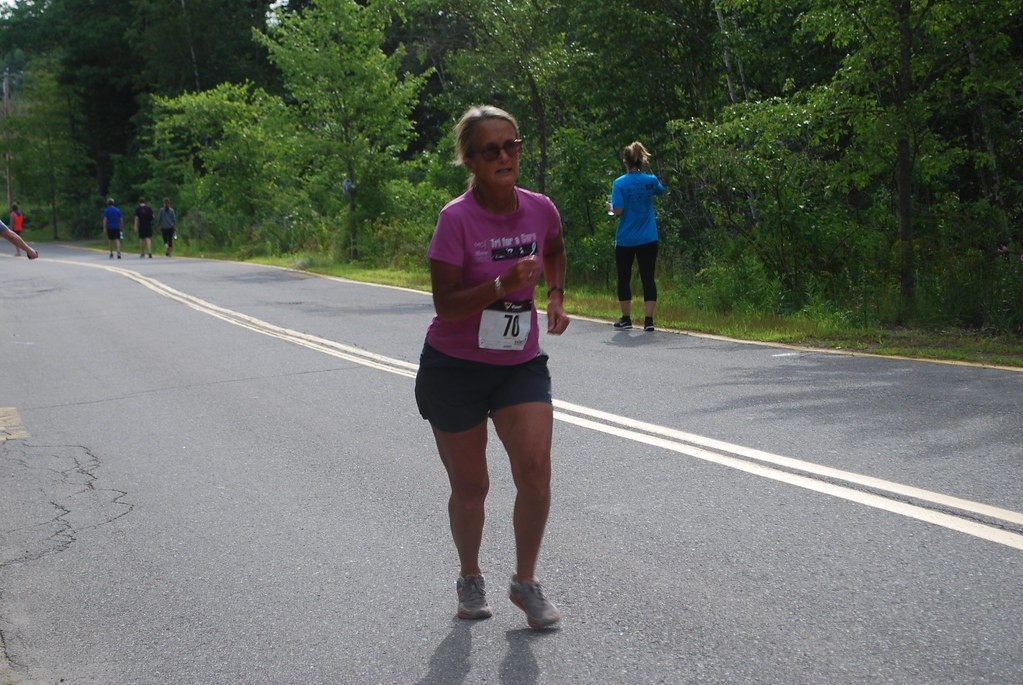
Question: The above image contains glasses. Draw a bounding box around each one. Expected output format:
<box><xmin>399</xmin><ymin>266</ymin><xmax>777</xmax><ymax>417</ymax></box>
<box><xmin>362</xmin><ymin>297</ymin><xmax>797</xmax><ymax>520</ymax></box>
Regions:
<box><xmin>471</xmin><ymin>139</ymin><xmax>523</xmax><ymax>161</ymax></box>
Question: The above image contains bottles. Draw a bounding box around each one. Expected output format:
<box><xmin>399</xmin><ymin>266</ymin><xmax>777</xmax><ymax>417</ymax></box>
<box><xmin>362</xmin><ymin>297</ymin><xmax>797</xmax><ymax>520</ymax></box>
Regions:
<box><xmin>120</xmin><ymin>231</ymin><xmax>123</xmax><ymax>239</ymax></box>
<box><xmin>606</xmin><ymin>195</ymin><xmax>614</xmax><ymax>215</ymax></box>
<box><xmin>174</xmin><ymin>231</ymin><xmax>177</xmax><ymax>239</ymax></box>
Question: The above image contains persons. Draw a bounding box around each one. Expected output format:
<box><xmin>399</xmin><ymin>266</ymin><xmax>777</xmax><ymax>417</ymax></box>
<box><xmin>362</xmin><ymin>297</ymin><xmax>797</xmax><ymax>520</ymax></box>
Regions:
<box><xmin>9</xmin><ymin>204</ymin><xmax>23</xmax><ymax>256</ymax></box>
<box><xmin>0</xmin><ymin>219</ymin><xmax>38</xmax><ymax>259</ymax></box>
<box><xmin>414</xmin><ymin>102</ymin><xmax>572</xmax><ymax>631</ymax></box>
<box><xmin>102</xmin><ymin>198</ymin><xmax>123</xmax><ymax>259</ymax></box>
<box><xmin>156</xmin><ymin>198</ymin><xmax>176</xmax><ymax>259</ymax></box>
<box><xmin>605</xmin><ymin>141</ymin><xmax>665</xmax><ymax>331</ymax></box>
<box><xmin>133</xmin><ymin>197</ymin><xmax>155</xmax><ymax>259</ymax></box>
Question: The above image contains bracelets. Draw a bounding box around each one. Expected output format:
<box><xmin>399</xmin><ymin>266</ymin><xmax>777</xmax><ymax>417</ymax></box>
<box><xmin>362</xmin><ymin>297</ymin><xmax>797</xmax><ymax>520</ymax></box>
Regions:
<box><xmin>547</xmin><ymin>286</ymin><xmax>564</xmax><ymax>298</ymax></box>
<box><xmin>495</xmin><ymin>275</ymin><xmax>506</xmax><ymax>298</ymax></box>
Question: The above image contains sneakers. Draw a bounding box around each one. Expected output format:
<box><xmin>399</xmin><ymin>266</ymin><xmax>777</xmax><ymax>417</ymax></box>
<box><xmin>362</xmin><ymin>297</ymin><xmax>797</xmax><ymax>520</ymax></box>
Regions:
<box><xmin>613</xmin><ymin>318</ymin><xmax>633</xmax><ymax>331</ymax></box>
<box><xmin>509</xmin><ymin>574</ymin><xmax>559</xmax><ymax>629</ymax></box>
<box><xmin>644</xmin><ymin>320</ymin><xmax>654</xmax><ymax>330</ymax></box>
<box><xmin>457</xmin><ymin>571</ymin><xmax>492</xmax><ymax>619</ymax></box>
